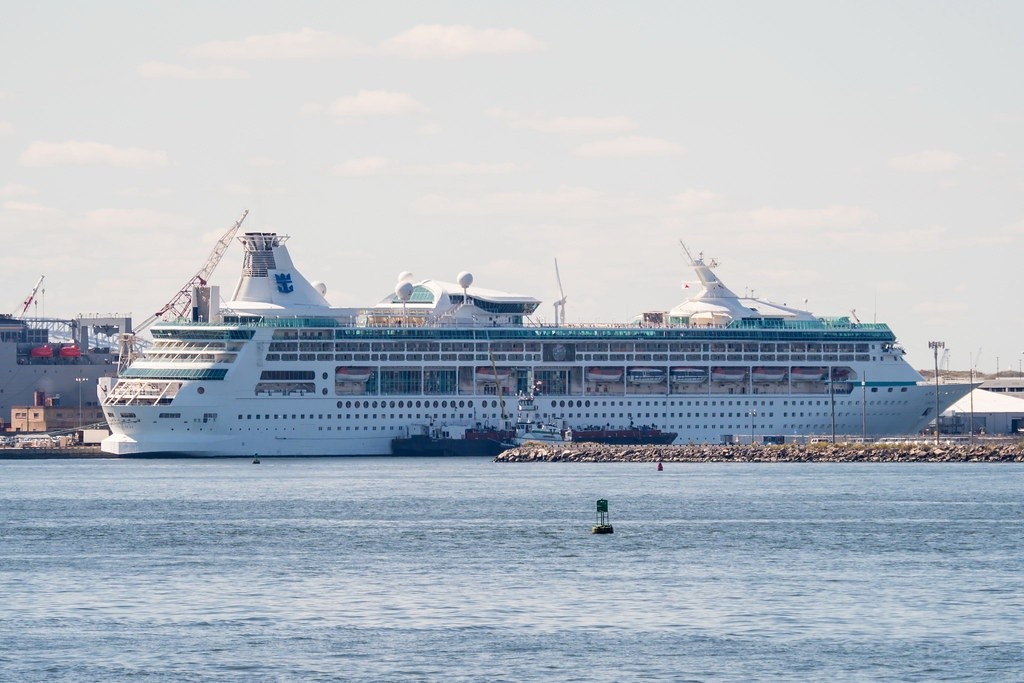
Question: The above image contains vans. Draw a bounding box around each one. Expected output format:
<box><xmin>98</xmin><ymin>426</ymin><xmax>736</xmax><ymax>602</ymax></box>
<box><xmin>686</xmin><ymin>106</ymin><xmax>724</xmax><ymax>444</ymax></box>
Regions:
<box><xmin>811</xmin><ymin>438</ymin><xmax>831</xmax><ymax>444</ymax></box>
<box><xmin>856</xmin><ymin>438</ymin><xmax>874</xmax><ymax>445</ymax></box>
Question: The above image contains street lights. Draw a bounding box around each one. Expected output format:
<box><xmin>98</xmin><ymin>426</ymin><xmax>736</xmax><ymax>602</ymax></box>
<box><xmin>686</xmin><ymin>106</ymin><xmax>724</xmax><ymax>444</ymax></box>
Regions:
<box><xmin>748</xmin><ymin>408</ymin><xmax>756</xmax><ymax>444</ymax></box>
<box><xmin>928</xmin><ymin>341</ymin><xmax>945</xmax><ymax>442</ymax></box>
<box><xmin>862</xmin><ymin>370</ymin><xmax>866</xmax><ymax>440</ymax></box>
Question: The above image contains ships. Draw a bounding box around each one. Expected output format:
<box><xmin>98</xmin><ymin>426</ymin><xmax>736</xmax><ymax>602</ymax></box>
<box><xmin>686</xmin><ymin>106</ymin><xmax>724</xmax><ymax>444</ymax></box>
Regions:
<box><xmin>0</xmin><ymin>274</ymin><xmax>131</xmax><ymax>427</ymax></box>
<box><xmin>95</xmin><ymin>209</ymin><xmax>987</xmax><ymax>458</ymax></box>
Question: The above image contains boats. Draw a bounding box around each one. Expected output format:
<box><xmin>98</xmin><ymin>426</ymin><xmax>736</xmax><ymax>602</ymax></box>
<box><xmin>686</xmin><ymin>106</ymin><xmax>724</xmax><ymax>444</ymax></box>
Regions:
<box><xmin>475</xmin><ymin>373</ymin><xmax>509</xmax><ymax>381</ymax></box>
<box><xmin>392</xmin><ymin>405</ymin><xmax>517</xmax><ymax>457</ymax></box>
<box><xmin>751</xmin><ymin>368</ymin><xmax>786</xmax><ymax>381</ymax></box>
<box><xmin>335</xmin><ymin>373</ymin><xmax>371</xmax><ymax>382</ymax></box>
<box><xmin>573</xmin><ymin>416</ymin><xmax>678</xmax><ymax>445</ymax></box>
<box><xmin>711</xmin><ymin>368</ymin><xmax>747</xmax><ymax>381</ymax></box>
<box><xmin>670</xmin><ymin>369</ymin><xmax>709</xmax><ymax>384</ymax></box>
<box><xmin>792</xmin><ymin>368</ymin><xmax>824</xmax><ymax>382</ymax></box>
<box><xmin>586</xmin><ymin>367</ymin><xmax>622</xmax><ymax>382</ymax></box>
<box><xmin>626</xmin><ymin>369</ymin><xmax>666</xmax><ymax>384</ymax></box>
<box><xmin>513</xmin><ymin>392</ymin><xmax>564</xmax><ymax>448</ymax></box>
